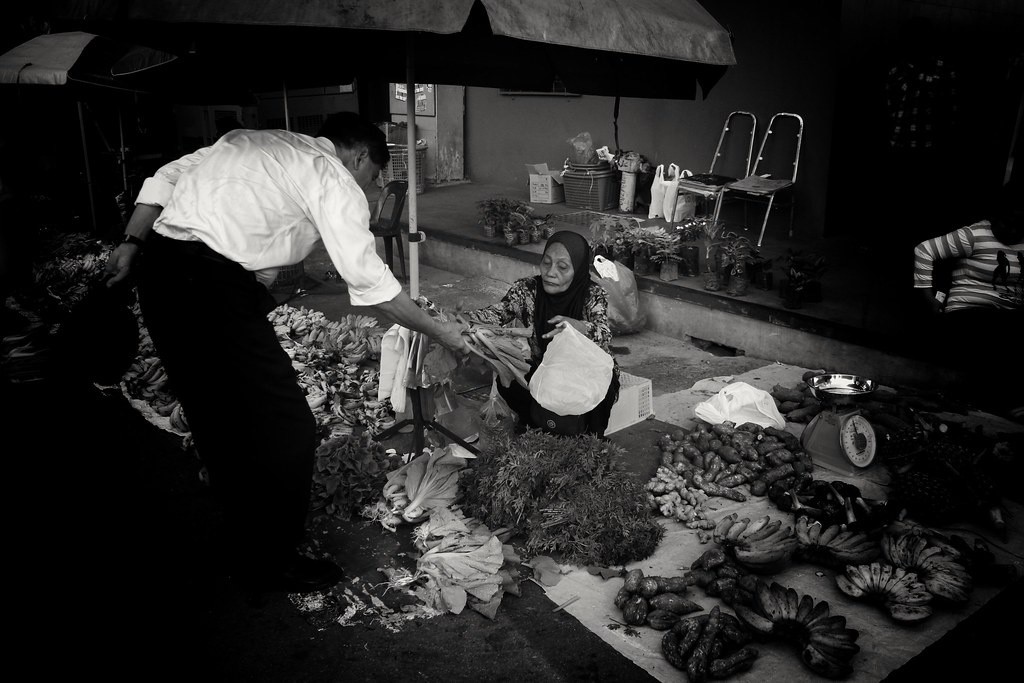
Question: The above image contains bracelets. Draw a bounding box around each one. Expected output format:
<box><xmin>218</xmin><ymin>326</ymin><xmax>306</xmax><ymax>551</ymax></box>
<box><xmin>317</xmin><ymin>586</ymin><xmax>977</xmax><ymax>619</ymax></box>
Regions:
<box><xmin>119</xmin><ymin>233</ymin><xmax>145</xmax><ymax>248</ymax></box>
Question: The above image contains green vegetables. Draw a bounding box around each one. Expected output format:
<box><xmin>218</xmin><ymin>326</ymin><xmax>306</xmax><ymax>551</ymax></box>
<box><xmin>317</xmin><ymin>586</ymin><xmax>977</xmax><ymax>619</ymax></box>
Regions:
<box><xmin>454</xmin><ymin>424</ymin><xmax>668</xmax><ymax>569</ymax></box>
<box><xmin>313</xmin><ymin>432</ymin><xmax>563</xmax><ymax>622</ymax></box>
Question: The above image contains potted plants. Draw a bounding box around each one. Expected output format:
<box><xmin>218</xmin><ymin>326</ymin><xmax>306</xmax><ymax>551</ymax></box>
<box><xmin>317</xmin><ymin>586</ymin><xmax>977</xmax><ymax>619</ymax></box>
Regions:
<box><xmin>475</xmin><ymin>199</ymin><xmax>556</xmax><ymax>247</ymax></box>
<box><xmin>588</xmin><ymin>215</ymin><xmax>827</xmax><ymax>310</ymax></box>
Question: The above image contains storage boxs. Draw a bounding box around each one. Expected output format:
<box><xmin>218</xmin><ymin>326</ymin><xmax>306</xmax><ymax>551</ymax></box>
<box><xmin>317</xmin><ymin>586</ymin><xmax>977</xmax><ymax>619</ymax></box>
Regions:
<box><xmin>604</xmin><ymin>370</ymin><xmax>653</xmax><ymax>436</ymax></box>
<box><xmin>560</xmin><ymin>159</ymin><xmax>620</xmax><ymax>211</ymax></box>
<box><xmin>524</xmin><ymin>163</ymin><xmax>565</xmax><ymax>204</ymax></box>
<box><xmin>377</xmin><ymin>143</ymin><xmax>428</xmax><ymax>197</ymax></box>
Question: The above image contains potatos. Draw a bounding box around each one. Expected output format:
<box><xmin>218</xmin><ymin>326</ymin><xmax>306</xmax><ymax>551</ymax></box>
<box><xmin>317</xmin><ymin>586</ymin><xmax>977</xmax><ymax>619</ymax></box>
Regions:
<box><xmin>658</xmin><ymin>420</ymin><xmax>814</xmax><ymax>502</ymax></box>
<box><xmin>662</xmin><ymin>604</ymin><xmax>759</xmax><ymax>682</ymax></box>
<box><xmin>614</xmin><ymin>568</ymin><xmax>704</xmax><ymax>631</ymax></box>
<box><xmin>770</xmin><ymin>371</ymin><xmax>824</xmax><ymax>423</ymax></box>
<box><xmin>683</xmin><ymin>549</ymin><xmax>761</xmax><ymax>595</ymax></box>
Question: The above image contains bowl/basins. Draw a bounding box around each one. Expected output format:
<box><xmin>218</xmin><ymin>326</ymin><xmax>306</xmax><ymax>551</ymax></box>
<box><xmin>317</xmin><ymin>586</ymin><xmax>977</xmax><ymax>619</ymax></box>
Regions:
<box><xmin>806</xmin><ymin>373</ymin><xmax>879</xmax><ymax>407</ymax></box>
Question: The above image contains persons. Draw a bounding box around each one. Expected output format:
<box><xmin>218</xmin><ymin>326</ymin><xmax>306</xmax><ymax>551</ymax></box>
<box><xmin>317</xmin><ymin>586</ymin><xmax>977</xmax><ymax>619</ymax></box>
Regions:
<box><xmin>100</xmin><ymin>117</ymin><xmax>472</xmax><ymax>610</ymax></box>
<box><xmin>412</xmin><ymin>231</ymin><xmax>621</xmax><ymax>442</ymax></box>
<box><xmin>913</xmin><ymin>180</ymin><xmax>1024</xmax><ymax>415</ymax></box>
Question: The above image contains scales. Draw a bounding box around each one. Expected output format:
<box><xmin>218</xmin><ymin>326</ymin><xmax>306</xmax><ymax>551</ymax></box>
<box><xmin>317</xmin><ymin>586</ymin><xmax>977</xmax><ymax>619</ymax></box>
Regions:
<box><xmin>798</xmin><ymin>371</ymin><xmax>889</xmax><ymax>477</ymax></box>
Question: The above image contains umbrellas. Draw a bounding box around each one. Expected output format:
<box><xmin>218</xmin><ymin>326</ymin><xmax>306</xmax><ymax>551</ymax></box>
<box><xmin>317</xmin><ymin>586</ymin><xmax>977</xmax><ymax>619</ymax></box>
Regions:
<box><xmin>0</xmin><ymin>30</ymin><xmax>219</xmax><ymax>232</ymax></box>
<box><xmin>82</xmin><ymin>0</ymin><xmax>738</xmax><ymax>456</ymax></box>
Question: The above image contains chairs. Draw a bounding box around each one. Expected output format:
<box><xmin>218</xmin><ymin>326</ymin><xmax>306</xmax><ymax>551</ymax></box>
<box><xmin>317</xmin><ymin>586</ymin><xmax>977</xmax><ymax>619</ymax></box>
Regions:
<box><xmin>336</xmin><ymin>180</ymin><xmax>409</xmax><ymax>284</ymax></box>
<box><xmin>669</xmin><ymin>111</ymin><xmax>756</xmax><ymax>234</ymax></box>
<box><xmin>714</xmin><ymin>113</ymin><xmax>803</xmax><ymax>246</ymax></box>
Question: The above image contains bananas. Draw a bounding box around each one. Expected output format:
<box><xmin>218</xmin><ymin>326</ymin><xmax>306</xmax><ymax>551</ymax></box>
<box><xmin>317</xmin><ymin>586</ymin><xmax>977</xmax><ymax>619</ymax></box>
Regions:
<box><xmin>264</xmin><ymin>303</ymin><xmax>397</xmax><ymax>438</ymax></box>
<box><xmin>713</xmin><ymin>512</ymin><xmax>972</xmax><ymax>676</ymax></box>
<box><xmin>124</xmin><ymin>355</ymin><xmax>190</xmax><ymax>432</ymax></box>
